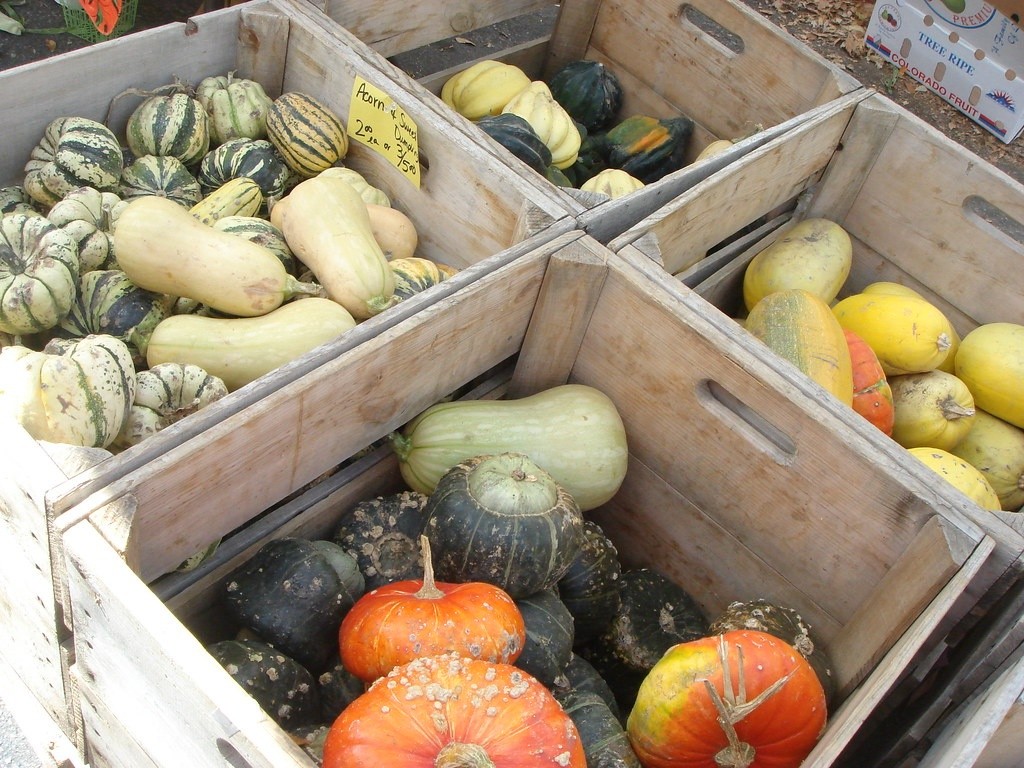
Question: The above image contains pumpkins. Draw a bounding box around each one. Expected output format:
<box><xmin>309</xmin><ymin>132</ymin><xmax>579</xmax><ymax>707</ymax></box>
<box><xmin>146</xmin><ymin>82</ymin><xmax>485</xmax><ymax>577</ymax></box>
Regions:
<box><xmin>0</xmin><ymin>57</ymin><xmax>1024</xmax><ymax>768</ymax></box>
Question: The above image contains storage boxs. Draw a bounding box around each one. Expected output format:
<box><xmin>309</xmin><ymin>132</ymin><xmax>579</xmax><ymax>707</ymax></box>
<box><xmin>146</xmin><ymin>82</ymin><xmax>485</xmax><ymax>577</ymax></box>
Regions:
<box><xmin>0</xmin><ymin>0</ymin><xmax>1024</xmax><ymax>768</ymax></box>
